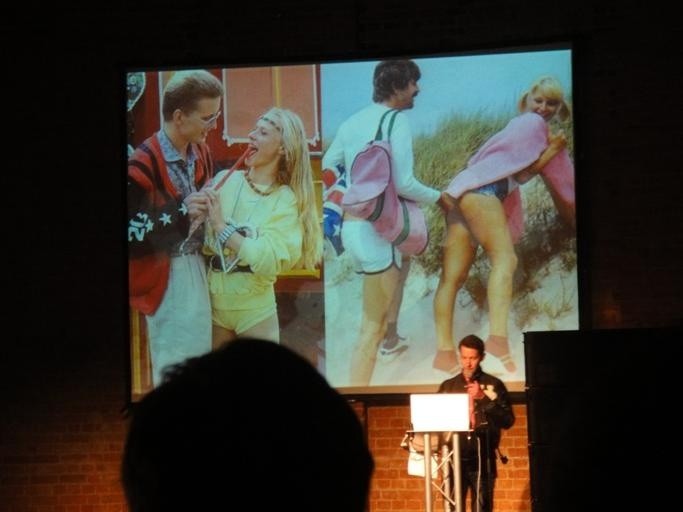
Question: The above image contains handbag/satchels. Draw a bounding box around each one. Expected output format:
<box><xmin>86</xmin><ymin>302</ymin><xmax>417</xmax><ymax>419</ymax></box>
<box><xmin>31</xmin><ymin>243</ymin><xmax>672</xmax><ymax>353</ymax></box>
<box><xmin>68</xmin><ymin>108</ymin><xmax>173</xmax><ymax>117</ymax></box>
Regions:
<box><xmin>338</xmin><ymin>108</ymin><xmax>433</xmax><ymax>257</ymax></box>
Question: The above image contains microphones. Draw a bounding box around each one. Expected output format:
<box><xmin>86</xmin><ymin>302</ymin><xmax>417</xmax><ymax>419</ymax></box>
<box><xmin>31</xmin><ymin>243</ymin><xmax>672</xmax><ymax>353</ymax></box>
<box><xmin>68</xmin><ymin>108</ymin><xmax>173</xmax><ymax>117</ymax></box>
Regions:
<box><xmin>462</xmin><ymin>367</ymin><xmax>474</xmax><ymax>383</ymax></box>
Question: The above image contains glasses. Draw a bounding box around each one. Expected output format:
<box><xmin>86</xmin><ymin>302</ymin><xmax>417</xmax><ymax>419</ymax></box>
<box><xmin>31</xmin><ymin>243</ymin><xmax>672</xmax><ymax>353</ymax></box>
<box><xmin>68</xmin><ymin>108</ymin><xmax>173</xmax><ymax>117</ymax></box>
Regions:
<box><xmin>194</xmin><ymin>111</ymin><xmax>223</xmax><ymax>128</ymax></box>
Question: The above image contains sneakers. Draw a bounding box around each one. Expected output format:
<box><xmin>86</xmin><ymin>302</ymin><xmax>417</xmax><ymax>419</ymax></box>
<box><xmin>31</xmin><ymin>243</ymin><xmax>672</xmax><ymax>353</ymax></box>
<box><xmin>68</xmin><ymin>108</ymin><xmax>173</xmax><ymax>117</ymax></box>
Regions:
<box><xmin>379</xmin><ymin>333</ymin><xmax>412</xmax><ymax>363</ymax></box>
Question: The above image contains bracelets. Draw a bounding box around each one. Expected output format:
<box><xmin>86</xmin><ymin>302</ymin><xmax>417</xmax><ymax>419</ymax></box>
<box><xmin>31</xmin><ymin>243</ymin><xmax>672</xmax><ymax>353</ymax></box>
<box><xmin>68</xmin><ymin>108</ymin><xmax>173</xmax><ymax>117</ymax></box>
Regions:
<box><xmin>218</xmin><ymin>224</ymin><xmax>237</xmax><ymax>245</ymax></box>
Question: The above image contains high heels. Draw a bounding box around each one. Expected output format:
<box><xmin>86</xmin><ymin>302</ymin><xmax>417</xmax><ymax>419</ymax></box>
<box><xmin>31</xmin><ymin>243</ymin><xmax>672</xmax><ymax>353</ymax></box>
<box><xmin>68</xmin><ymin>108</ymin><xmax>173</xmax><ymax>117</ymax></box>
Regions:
<box><xmin>478</xmin><ymin>346</ymin><xmax>525</xmax><ymax>380</ymax></box>
<box><xmin>431</xmin><ymin>363</ymin><xmax>465</xmax><ymax>384</ymax></box>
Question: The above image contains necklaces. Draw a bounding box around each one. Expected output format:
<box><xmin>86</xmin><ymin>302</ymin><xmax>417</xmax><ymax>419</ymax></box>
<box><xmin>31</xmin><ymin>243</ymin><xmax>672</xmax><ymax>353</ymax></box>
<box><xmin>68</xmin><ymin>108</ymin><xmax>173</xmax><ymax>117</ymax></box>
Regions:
<box><xmin>243</xmin><ymin>170</ymin><xmax>285</xmax><ymax>196</ymax></box>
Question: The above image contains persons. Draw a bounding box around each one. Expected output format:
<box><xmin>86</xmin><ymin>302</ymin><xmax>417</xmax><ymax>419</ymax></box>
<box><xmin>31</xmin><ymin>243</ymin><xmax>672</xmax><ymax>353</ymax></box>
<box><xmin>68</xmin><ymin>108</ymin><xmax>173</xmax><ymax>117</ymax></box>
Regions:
<box><xmin>436</xmin><ymin>335</ymin><xmax>514</xmax><ymax>512</ymax></box>
<box><xmin>128</xmin><ymin>69</ymin><xmax>224</xmax><ymax>388</ymax></box>
<box><xmin>202</xmin><ymin>109</ymin><xmax>323</xmax><ymax>351</ymax></box>
<box><xmin>120</xmin><ymin>338</ymin><xmax>373</xmax><ymax>512</ymax></box>
<box><xmin>322</xmin><ymin>59</ymin><xmax>457</xmax><ymax>387</ymax></box>
<box><xmin>433</xmin><ymin>78</ymin><xmax>574</xmax><ymax>374</ymax></box>
